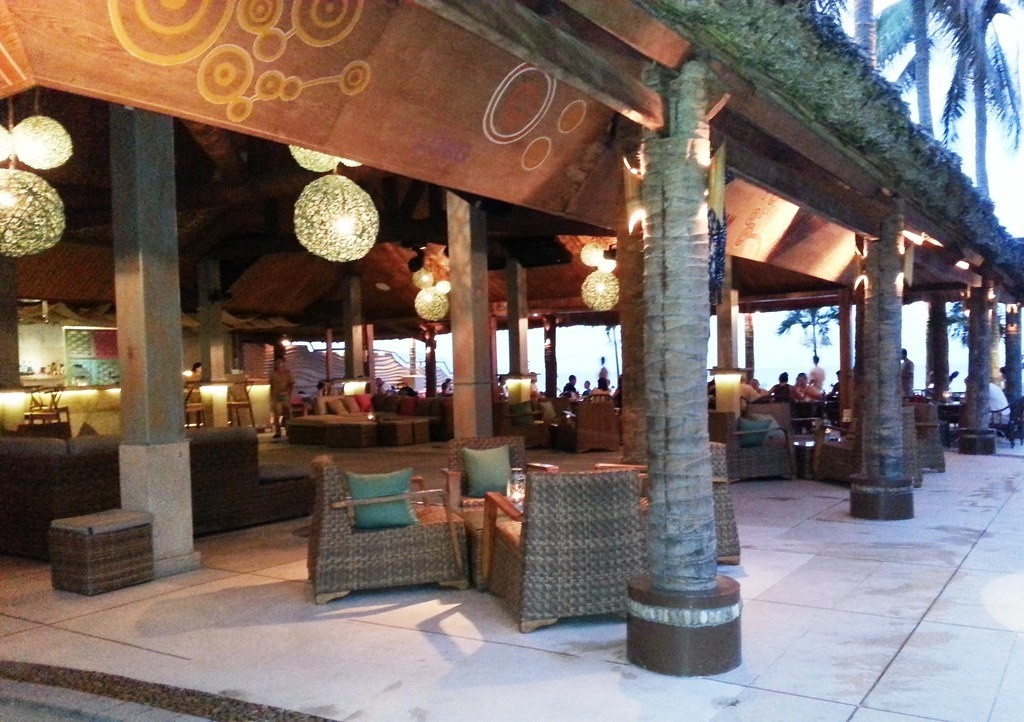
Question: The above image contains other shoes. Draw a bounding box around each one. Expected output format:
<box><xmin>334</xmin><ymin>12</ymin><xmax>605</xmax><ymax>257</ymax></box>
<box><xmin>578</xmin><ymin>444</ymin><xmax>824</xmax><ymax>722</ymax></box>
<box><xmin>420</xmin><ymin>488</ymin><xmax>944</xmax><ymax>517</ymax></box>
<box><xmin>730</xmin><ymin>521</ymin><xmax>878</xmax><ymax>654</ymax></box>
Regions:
<box><xmin>273</xmin><ymin>434</ymin><xmax>282</xmax><ymax>438</ymax></box>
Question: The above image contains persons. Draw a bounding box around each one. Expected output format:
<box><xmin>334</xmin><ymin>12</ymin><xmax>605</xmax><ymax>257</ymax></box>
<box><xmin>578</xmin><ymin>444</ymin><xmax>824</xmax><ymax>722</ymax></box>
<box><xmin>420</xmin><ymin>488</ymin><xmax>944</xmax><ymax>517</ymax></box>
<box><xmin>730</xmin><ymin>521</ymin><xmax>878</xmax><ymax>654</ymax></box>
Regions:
<box><xmin>563</xmin><ymin>373</ymin><xmax>577</xmax><ymax>393</ymax></box>
<box><xmin>596</xmin><ymin>367</ymin><xmax>622</xmax><ymax>408</ymax></box>
<box><xmin>963</xmin><ymin>375</ymin><xmax>1010</xmax><ymax>425</ymax></box>
<box><xmin>269</xmin><ymin>355</ymin><xmax>295</xmax><ymax>438</ymax></box>
<box><xmin>809</xmin><ymin>356</ymin><xmax>825</xmax><ymax>389</ymax></box>
<box><xmin>825</xmin><ymin>369</ymin><xmax>842</xmax><ymax>426</ymax></box>
<box><xmin>582</xmin><ymin>380</ymin><xmax>591</xmax><ymax>396</ymax></box>
<box><xmin>707</xmin><ymin>370</ymin><xmax>825</xmax><ymax>435</ymax></box>
<box><xmin>530</xmin><ymin>371</ymin><xmax>547</xmax><ymax>405</ymax></box>
<box><xmin>496</xmin><ymin>374</ymin><xmax>508</xmax><ymax>402</ymax></box>
<box><xmin>901</xmin><ymin>347</ymin><xmax>915</xmax><ymax>396</ymax></box>
<box><xmin>1000</xmin><ymin>366</ymin><xmax>1007</xmax><ymax>394</ymax></box>
<box><xmin>440</xmin><ymin>378</ymin><xmax>454</xmax><ymax>394</ymax></box>
<box><xmin>192</xmin><ymin>361</ymin><xmax>196</xmax><ymax>376</ymax></box>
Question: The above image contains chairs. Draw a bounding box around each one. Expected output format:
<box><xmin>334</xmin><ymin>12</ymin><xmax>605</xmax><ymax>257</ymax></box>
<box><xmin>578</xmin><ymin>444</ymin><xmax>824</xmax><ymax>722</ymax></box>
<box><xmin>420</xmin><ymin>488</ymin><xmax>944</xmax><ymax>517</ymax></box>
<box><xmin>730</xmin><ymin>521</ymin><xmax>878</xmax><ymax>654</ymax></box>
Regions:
<box><xmin>494</xmin><ymin>395</ymin><xmax>621</xmax><ymax>451</ymax></box>
<box><xmin>710</xmin><ymin>402</ymin><xmax>947</xmax><ymax>489</ymax></box>
<box><xmin>227</xmin><ymin>386</ymin><xmax>255</xmax><ymax>427</ymax></box>
<box><xmin>27</xmin><ymin>386</ymin><xmax>69</xmax><ymax>425</ymax></box>
<box><xmin>990</xmin><ymin>396</ymin><xmax>1024</xmax><ymax>448</ymax></box>
<box><xmin>185</xmin><ymin>386</ymin><xmax>206</xmax><ymax>427</ymax></box>
<box><xmin>310</xmin><ymin>439</ymin><xmax>743</xmax><ymax>631</ymax></box>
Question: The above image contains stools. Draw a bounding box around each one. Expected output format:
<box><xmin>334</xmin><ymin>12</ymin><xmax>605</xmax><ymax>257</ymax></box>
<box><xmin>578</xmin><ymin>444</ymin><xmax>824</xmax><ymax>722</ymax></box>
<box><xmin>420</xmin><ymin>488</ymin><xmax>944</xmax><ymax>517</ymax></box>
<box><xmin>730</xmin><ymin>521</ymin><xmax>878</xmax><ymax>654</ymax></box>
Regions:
<box><xmin>50</xmin><ymin>509</ymin><xmax>156</xmax><ymax>594</ymax></box>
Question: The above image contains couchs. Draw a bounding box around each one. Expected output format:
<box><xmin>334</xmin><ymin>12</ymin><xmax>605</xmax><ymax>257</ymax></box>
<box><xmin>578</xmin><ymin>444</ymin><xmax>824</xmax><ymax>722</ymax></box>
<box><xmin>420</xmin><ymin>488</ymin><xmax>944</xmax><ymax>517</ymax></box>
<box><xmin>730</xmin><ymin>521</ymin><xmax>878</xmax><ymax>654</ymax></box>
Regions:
<box><xmin>1</xmin><ymin>429</ymin><xmax>311</xmax><ymax>558</ymax></box>
<box><xmin>290</xmin><ymin>395</ymin><xmax>454</xmax><ymax>447</ymax></box>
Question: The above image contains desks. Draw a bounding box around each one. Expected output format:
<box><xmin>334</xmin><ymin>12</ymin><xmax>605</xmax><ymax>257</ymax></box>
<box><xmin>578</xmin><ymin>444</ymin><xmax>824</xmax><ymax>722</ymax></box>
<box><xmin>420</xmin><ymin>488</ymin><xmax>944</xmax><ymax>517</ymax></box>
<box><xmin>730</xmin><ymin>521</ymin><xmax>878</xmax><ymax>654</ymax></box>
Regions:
<box><xmin>941</xmin><ymin>401</ymin><xmax>966</xmax><ymax>443</ymax></box>
<box><xmin>459</xmin><ymin>510</ymin><xmax>489</xmax><ymax>590</ymax></box>
<box><xmin>790</xmin><ymin>439</ymin><xmax>815</xmax><ymax>478</ymax></box>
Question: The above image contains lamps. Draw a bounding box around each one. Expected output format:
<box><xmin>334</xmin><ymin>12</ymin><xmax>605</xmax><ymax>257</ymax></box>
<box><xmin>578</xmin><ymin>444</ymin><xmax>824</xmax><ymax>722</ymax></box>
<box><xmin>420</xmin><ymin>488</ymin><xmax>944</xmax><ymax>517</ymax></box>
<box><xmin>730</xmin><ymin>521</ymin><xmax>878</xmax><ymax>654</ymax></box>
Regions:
<box><xmin>410</xmin><ymin>270</ymin><xmax>451</xmax><ymax>320</ymax></box>
<box><xmin>285</xmin><ymin>140</ymin><xmax>380</xmax><ymax>262</ymax></box>
<box><xmin>508</xmin><ymin>475</ymin><xmax>526</xmax><ymax>507</ymax></box>
<box><xmin>581</xmin><ymin>243</ymin><xmax>620</xmax><ymax>309</ymax></box>
<box><xmin>0</xmin><ymin>115</ymin><xmax>73</xmax><ymax>257</ymax></box>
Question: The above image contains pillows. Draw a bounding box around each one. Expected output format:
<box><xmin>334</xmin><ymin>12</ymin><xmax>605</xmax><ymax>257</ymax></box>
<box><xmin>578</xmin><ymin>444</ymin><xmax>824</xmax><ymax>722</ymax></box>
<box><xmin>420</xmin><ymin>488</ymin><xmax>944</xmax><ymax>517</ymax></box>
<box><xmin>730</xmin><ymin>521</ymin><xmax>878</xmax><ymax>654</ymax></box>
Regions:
<box><xmin>459</xmin><ymin>446</ymin><xmax>510</xmax><ymax>496</ymax></box>
<box><xmin>510</xmin><ymin>400</ymin><xmax>533</xmax><ymax>426</ymax></box>
<box><xmin>329</xmin><ymin>396</ymin><xmax>441</xmax><ymax>415</ymax></box>
<box><xmin>343</xmin><ymin>469</ymin><xmax>419</xmax><ymax>529</ymax></box>
<box><xmin>553</xmin><ymin>429</ymin><xmax>577</xmax><ymax>450</ymax></box>
<box><xmin>740</xmin><ymin>416</ymin><xmax>770</xmax><ymax>448</ymax></box>
